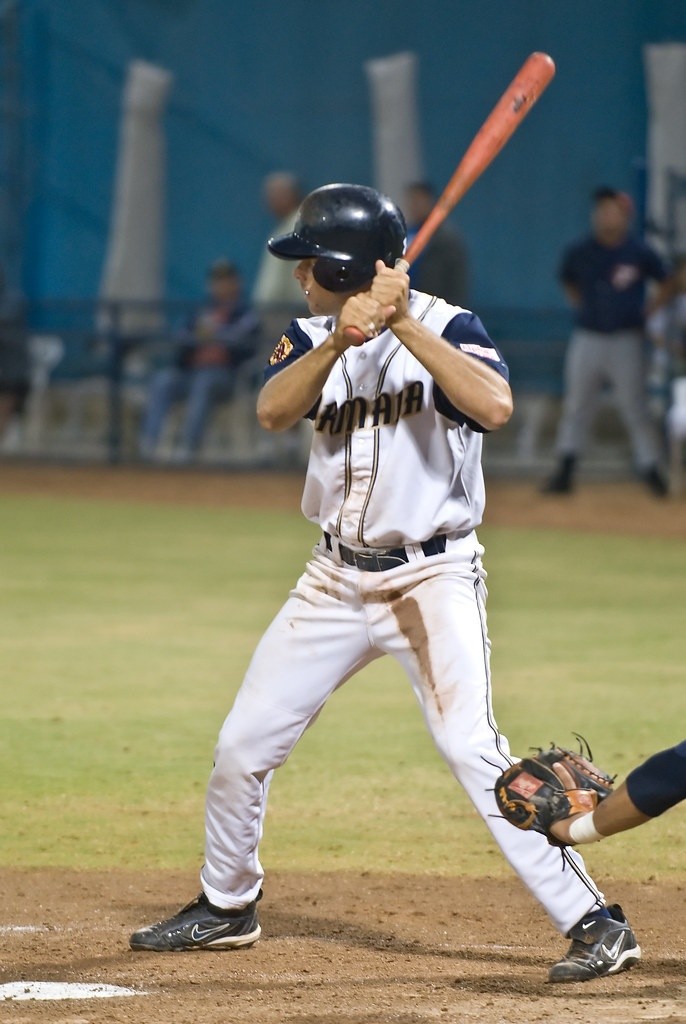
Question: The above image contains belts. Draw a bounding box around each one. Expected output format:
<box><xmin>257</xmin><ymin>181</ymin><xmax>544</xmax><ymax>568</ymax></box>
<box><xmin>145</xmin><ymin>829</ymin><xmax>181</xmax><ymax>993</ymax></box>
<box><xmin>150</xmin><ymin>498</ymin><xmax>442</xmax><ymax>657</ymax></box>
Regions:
<box><xmin>323</xmin><ymin>528</ymin><xmax>447</xmax><ymax>572</ymax></box>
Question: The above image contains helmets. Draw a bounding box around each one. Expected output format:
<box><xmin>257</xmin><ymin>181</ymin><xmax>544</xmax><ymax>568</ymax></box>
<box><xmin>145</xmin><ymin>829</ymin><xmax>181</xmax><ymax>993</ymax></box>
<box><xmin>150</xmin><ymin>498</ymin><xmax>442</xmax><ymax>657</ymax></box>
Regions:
<box><xmin>263</xmin><ymin>182</ymin><xmax>411</xmax><ymax>296</ymax></box>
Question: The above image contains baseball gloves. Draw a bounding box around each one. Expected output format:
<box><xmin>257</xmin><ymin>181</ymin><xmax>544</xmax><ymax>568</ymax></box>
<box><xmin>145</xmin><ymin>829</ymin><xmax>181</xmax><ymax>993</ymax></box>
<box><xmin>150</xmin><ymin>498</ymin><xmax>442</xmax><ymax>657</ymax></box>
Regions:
<box><xmin>492</xmin><ymin>748</ymin><xmax>616</xmax><ymax>847</ymax></box>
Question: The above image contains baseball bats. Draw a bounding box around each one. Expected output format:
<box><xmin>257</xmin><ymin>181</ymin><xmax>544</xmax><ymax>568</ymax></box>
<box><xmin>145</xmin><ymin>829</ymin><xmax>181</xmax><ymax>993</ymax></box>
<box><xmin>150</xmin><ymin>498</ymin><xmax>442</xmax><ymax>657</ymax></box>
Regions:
<box><xmin>342</xmin><ymin>47</ymin><xmax>554</xmax><ymax>347</ymax></box>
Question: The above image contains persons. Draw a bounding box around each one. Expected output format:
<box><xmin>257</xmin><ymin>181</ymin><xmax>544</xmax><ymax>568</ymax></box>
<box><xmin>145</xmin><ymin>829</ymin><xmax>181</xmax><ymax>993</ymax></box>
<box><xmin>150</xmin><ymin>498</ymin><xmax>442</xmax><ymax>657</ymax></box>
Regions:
<box><xmin>493</xmin><ymin>737</ymin><xmax>686</xmax><ymax>846</ymax></box>
<box><xmin>0</xmin><ymin>174</ymin><xmax>686</xmax><ymax>497</ymax></box>
<box><xmin>130</xmin><ymin>187</ymin><xmax>641</xmax><ymax>981</ymax></box>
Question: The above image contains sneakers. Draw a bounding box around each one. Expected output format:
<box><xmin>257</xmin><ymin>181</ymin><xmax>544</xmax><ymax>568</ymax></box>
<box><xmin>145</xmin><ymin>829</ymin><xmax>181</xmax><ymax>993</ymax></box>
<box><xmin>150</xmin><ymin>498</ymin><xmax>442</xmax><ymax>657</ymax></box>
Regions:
<box><xmin>546</xmin><ymin>904</ymin><xmax>642</xmax><ymax>985</ymax></box>
<box><xmin>129</xmin><ymin>888</ymin><xmax>265</xmax><ymax>953</ymax></box>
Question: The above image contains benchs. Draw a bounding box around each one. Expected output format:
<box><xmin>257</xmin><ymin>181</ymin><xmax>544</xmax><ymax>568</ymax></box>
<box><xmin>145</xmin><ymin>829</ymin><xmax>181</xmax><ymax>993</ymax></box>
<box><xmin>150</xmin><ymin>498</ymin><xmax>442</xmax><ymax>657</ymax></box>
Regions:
<box><xmin>28</xmin><ymin>334</ymin><xmax>312</xmax><ymax>471</ymax></box>
